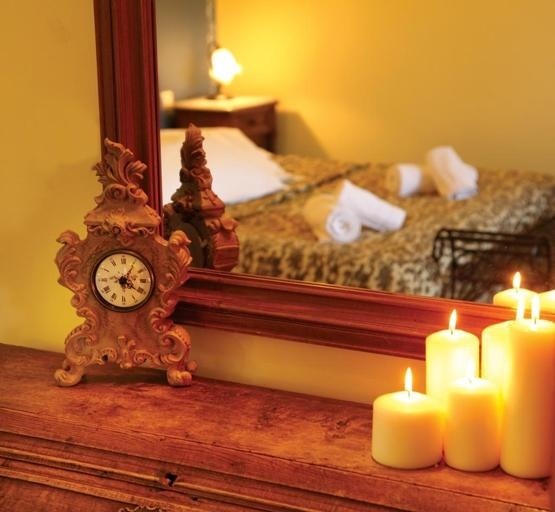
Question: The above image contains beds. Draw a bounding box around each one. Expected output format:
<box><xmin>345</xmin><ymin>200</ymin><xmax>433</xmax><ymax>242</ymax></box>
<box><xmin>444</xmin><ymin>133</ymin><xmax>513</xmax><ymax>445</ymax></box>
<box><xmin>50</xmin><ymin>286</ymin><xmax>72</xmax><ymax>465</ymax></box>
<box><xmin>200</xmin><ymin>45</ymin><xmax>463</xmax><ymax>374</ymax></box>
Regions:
<box><xmin>159</xmin><ymin>123</ymin><xmax>554</xmax><ymax>302</ymax></box>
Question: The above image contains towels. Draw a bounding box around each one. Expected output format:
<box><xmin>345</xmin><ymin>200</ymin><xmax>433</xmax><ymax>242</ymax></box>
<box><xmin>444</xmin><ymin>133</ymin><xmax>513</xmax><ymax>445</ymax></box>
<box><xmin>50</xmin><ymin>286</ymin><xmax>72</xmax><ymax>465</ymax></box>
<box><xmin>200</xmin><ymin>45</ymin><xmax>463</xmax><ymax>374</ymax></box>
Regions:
<box><xmin>304</xmin><ymin>195</ymin><xmax>362</xmax><ymax>244</ymax></box>
<box><xmin>429</xmin><ymin>145</ymin><xmax>478</xmax><ymax>201</ymax></box>
<box><xmin>384</xmin><ymin>163</ymin><xmax>477</xmax><ymax>197</ymax></box>
<box><xmin>332</xmin><ymin>178</ymin><xmax>406</xmax><ymax>232</ymax></box>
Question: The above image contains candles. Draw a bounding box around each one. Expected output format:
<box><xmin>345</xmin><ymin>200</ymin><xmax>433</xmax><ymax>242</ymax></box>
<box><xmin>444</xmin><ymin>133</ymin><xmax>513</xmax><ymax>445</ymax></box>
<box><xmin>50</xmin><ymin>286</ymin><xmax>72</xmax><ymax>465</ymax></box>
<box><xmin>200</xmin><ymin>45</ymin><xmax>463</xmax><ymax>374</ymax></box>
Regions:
<box><xmin>371</xmin><ymin>368</ymin><xmax>443</xmax><ymax>469</ymax></box>
<box><xmin>539</xmin><ymin>290</ymin><xmax>555</xmax><ymax>314</ymax></box>
<box><xmin>443</xmin><ymin>363</ymin><xmax>501</xmax><ymax>472</ymax></box>
<box><xmin>425</xmin><ymin>309</ymin><xmax>480</xmax><ymax>396</ymax></box>
<box><xmin>493</xmin><ymin>272</ymin><xmax>539</xmax><ymax>310</ymax></box>
<box><xmin>482</xmin><ymin>294</ymin><xmax>555</xmax><ymax>479</ymax></box>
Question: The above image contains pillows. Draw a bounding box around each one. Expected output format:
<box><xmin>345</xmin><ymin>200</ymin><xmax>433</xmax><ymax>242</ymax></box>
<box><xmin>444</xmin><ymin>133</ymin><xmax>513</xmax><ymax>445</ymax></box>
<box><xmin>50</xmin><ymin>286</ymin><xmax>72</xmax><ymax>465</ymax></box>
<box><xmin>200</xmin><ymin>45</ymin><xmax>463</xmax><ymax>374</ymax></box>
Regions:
<box><xmin>160</xmin><ymin>127</ymin><xmax>287</xmax><ymax>205</ymax></box>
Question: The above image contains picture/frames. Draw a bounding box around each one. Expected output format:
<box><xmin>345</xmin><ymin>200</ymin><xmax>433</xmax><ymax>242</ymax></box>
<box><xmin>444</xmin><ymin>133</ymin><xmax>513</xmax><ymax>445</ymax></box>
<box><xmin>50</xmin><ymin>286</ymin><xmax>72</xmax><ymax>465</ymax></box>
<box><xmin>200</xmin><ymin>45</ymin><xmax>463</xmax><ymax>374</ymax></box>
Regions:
<box><xmin>94</xmin><ymin>1</ymin><xmax>555</xmax><ymax>361</ymax></box>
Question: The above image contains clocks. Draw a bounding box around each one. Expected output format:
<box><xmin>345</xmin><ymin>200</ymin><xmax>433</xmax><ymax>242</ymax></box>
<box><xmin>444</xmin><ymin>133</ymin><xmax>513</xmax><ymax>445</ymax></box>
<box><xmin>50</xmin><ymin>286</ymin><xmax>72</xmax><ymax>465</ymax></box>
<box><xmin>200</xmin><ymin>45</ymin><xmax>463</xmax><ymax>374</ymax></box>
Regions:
<box><xmin>54</xmin><ymin>138</ymin><xmax>198</xmax><ymax>387</ymax></box>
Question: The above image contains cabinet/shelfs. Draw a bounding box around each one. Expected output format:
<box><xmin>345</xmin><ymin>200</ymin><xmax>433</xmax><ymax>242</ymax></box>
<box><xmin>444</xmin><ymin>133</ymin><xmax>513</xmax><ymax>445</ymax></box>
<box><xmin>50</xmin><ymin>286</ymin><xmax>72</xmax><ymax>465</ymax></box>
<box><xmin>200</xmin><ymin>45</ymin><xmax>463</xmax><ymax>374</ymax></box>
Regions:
<box><xmin>0</xmin><ymin>343</ymin><xmax>555</xmax><ymax>512</ymax></box>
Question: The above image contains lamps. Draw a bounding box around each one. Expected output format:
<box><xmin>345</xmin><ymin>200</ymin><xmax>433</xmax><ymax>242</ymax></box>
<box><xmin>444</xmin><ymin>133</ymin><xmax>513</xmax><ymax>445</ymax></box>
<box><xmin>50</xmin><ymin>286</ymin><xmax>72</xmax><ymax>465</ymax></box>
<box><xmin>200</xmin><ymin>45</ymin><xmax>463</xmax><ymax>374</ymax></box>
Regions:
<box><xmin>206</xmin><ymin>40</ymin><xmax>239</xmax><ymax>99</ymax></box>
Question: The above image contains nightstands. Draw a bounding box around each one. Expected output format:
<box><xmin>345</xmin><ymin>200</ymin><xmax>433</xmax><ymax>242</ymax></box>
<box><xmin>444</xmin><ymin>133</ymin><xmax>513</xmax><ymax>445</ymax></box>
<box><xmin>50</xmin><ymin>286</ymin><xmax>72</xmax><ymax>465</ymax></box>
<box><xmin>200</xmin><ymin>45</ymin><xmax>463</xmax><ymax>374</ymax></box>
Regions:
<box><xmin>173</xmin><ymin>97</ymin><xmax>279</xmax><ymax>154</ymax></box>
